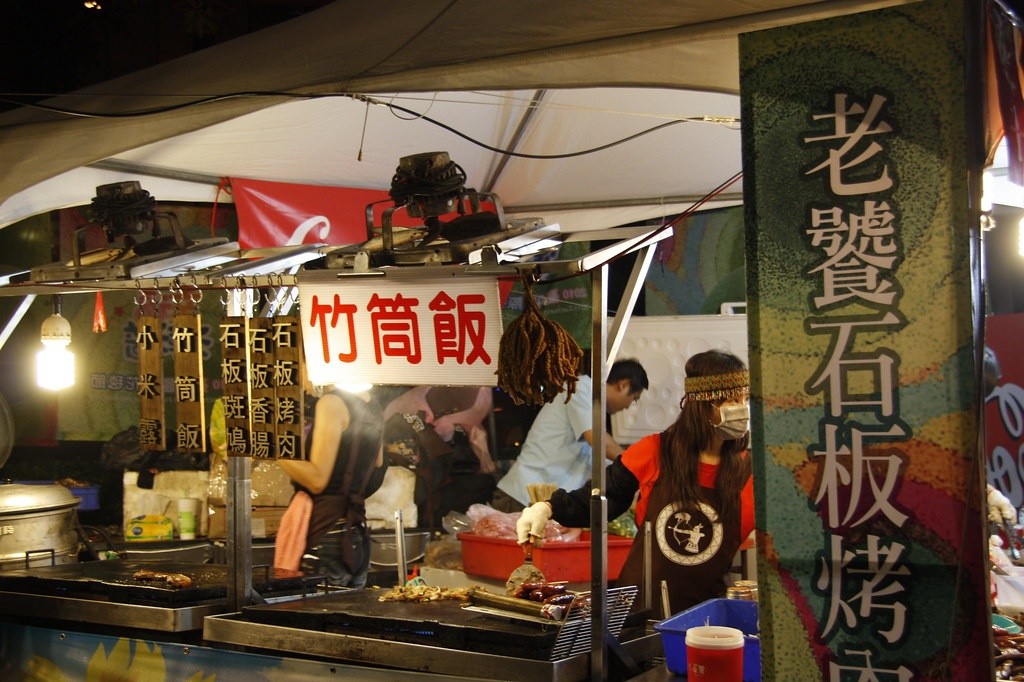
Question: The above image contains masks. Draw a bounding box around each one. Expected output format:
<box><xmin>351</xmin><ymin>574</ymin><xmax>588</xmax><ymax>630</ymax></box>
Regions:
<box><xmin>711</xmin><ymin>404</ymin><xmax>750</xmax><ymax>440</ymax></box>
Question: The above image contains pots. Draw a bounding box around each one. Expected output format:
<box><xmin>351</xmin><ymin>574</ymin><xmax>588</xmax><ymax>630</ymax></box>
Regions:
<box><xmin>0</xmin><ymin>479</ymin><xmax>85</xmax><ymax>571</ymax></box>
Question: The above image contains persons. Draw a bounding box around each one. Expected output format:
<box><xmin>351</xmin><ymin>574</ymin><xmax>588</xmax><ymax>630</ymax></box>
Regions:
<box><xmin>517</xmin><ymin>349</ymin><xmax>754</xmax><ymax>627</ymax></box>
<box><xmin>273</xmin><ymin>388</ymin><xmax>385</xmax><ymax>591</ymax></box>
<box><xmin>413</xmin><ymin>387</ymin><xmax>492</xmax><ymax>531</ymax></box>
<box><xmin>495</xmin><ymin>357</ymin><xmax>650</xmax><ymax>514</ymax></box>
<box><xmin>984</xmin><ymin>345</ymin><xmax>1016</xmax><ymax>529</ymax></box>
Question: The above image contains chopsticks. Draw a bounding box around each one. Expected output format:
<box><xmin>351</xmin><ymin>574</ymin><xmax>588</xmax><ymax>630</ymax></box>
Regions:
<box><xmin>528</xmin><ymin>484</ymin><xmax>557</xmax><ymax>502</ymax></box>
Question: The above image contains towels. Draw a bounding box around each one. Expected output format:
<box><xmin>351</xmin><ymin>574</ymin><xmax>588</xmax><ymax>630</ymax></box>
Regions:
<box><xmin>273</xmin><ymin>491</ymin><xmax>313</xmax><ymax>570</ymax></box>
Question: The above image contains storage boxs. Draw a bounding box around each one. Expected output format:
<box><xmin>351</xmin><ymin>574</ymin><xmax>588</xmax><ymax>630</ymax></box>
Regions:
<box><xmin>11</xmin><ymin>480</ymin><xmax>100</xmax><ymax>510</ymax></box>
<box><xmin>653</xmin><ymin>597</ymin><xmax>761</xmax><ymax>682</ymax></box>
<box><xmin>455</xmin><ymin>529</ymin><xmax>634</xmax><ymax>584</ymax></box>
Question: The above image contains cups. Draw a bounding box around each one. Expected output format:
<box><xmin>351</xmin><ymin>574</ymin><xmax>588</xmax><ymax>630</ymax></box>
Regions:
<box><xmin>177</xmin><ymin>498</ymin><xmax>197</xmax><ymax>541</ymax></box>
<box><xmin>686</xmin><ymin>626</ymin><xmax>745</xmax><ymax>682</ymax></box>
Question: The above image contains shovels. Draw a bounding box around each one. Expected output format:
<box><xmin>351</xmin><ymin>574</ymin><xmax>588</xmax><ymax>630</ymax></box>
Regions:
<box><xmin>506</xmin><ymin>537</ymin><xmax>548</xmax><ymax>595</ymax></box>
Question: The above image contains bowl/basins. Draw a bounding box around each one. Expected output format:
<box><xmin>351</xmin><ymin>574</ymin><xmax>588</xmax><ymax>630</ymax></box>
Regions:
<box><xmin>457</xmin><ymin>526</ymin><xmax>637</xmax><ymax>582</ymax></box>
<box><xmin>652</xmin><ymin>598</ymin><xmax>761</xmax><ymax>682</ymax></box>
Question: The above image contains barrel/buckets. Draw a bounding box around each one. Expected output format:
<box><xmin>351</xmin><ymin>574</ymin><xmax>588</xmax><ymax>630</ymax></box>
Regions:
<box><xmin>368</xmin><ymin>532</ymin><xmax>429</xmax><ymax>570</ymax></box>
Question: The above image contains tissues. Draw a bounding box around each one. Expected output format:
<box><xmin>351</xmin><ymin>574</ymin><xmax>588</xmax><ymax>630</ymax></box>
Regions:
<box><xmin>125</xmin><ymin>491</ymin><xmax>173</xmax><ymax>541</ymax></box>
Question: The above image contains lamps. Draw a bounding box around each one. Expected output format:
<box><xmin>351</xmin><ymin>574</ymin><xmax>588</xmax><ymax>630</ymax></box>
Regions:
<box><xmin>37</xmin><ymin>297</ymin><xmax>74</xmax><ymax>391</ymax></box>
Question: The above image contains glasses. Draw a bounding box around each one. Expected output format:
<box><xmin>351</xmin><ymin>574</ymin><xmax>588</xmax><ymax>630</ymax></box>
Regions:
<box><xmin>629</xmin><ymin>386</ymin><xmax>637</xmax><ymax>405</ymax></box>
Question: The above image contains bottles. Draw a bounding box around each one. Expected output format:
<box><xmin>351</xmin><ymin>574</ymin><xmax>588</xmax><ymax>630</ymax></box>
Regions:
<box><xmin>726</xmin><ymin>580</ymin><xmax>758</xmax><ymax>601</ymax></box>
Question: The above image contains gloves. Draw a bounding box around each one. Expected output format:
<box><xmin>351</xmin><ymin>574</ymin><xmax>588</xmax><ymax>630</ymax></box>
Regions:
<box><xmin>986</xmin><ymin>483</ymin><xmax>1017</xmax><ymax>531</ymax></box>
